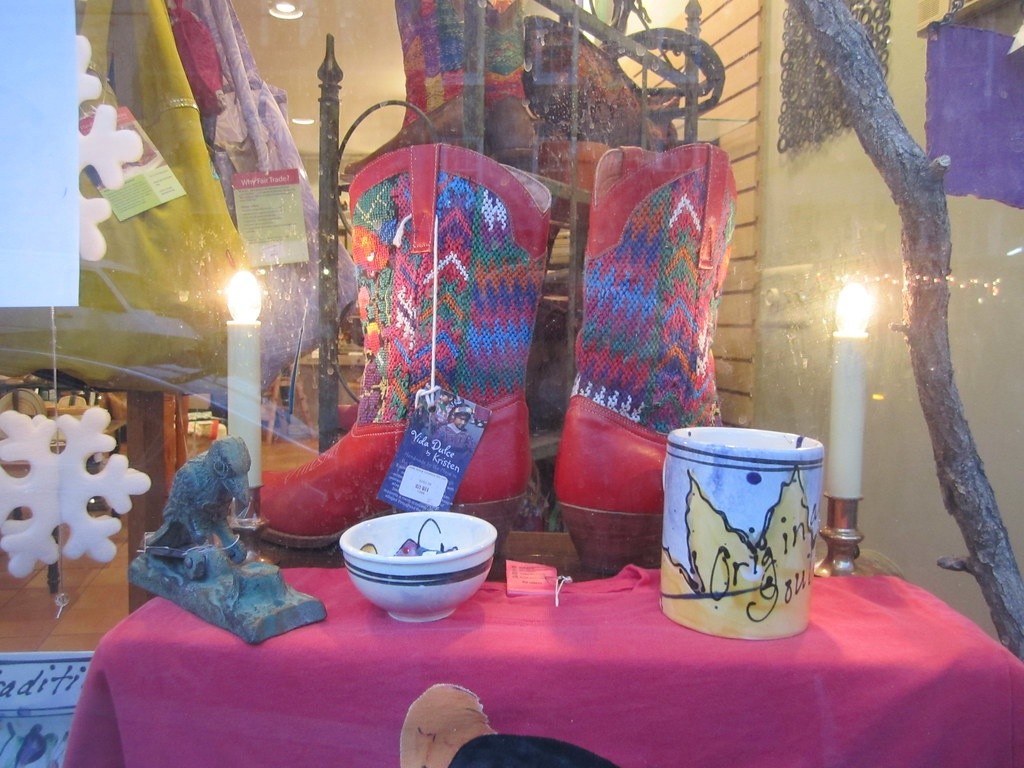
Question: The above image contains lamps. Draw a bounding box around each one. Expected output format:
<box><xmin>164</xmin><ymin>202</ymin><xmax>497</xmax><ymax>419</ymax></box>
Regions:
<box><xmin>825</xmin><ymin>281</ymin><xmax>875</xmax><ymax>497</ymax></box>
<box><xmin>225</xmin><ymin>269</ymin><xmax>262</xmax><ymax>486</ymax></box>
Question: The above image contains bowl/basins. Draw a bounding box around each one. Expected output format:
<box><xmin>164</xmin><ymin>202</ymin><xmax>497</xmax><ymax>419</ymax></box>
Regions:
<box><xmin>338</xmin><ymin>512</ymin><xmax>497</xmax><ymax>624</ymax></box>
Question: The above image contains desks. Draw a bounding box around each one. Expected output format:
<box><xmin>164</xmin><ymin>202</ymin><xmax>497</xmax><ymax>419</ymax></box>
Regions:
<box><xmin>61</xmin><ymin>533</ymin><xmax>1023</xmax><ymax>768</ymax></box>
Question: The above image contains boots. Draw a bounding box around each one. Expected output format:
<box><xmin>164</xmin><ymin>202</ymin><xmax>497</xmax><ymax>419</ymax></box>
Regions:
<box><xmin>555</xmin><ymin>143</ymin><xmax>736</xmax><ymax>574</ymax></box>
<box><xmin>258</xmin><ymin>143</ymin><xmax>550</xmax><ymax>548</ymax></box>
<box><xmin>340</xmin><ymin>0</ymin><xmax>539</xmax><ymax>182</ymax></box>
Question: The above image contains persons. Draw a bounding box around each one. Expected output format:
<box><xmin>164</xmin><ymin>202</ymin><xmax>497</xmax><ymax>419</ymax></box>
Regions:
<box><xmin>429</xmin><ymin>390</ymin><xmax>472</xmax><ymax>458</ymax></box>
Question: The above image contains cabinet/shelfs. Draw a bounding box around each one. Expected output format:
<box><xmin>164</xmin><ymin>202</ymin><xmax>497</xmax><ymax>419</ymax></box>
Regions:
<box><xmin>317</xmin><ymin>0</ymin><xmax>703</xmax><ymax>464</ymax></box>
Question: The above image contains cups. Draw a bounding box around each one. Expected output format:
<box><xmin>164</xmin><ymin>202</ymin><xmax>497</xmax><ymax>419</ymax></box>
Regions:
<box><xmin>657</xmin><ymin>426</ymin><xmax>824</xmax><ymax>640</ymax></box>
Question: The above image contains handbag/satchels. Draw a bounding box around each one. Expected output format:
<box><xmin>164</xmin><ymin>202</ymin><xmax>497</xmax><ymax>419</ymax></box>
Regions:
<box><xmin>1</xmin><ymin>0</ymin><xmax>360</xmax><ymax>392</ymax></box>
